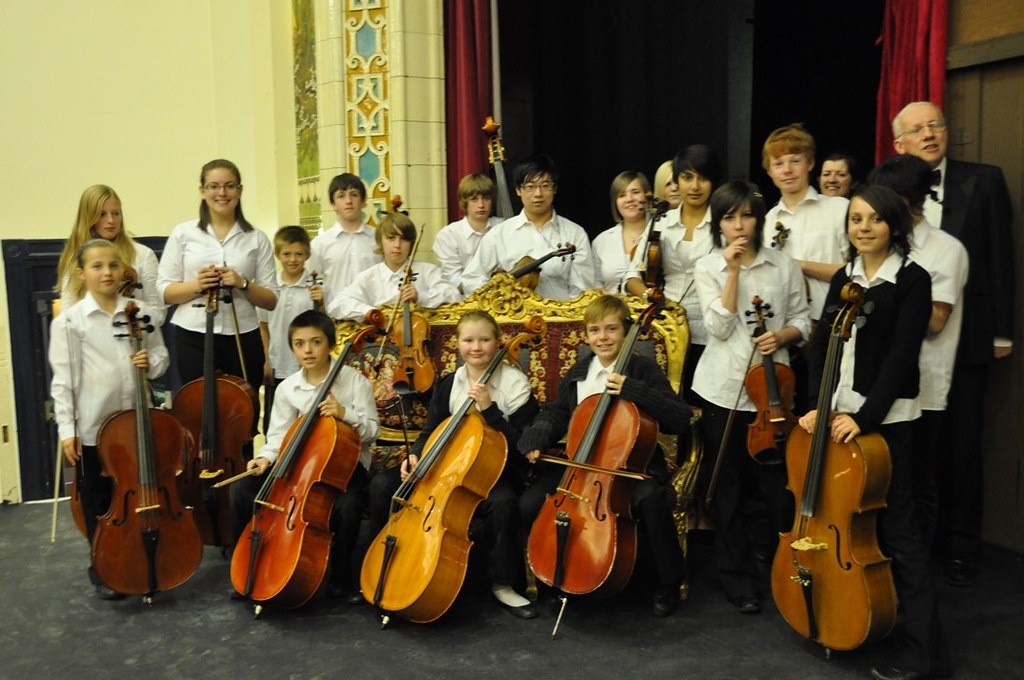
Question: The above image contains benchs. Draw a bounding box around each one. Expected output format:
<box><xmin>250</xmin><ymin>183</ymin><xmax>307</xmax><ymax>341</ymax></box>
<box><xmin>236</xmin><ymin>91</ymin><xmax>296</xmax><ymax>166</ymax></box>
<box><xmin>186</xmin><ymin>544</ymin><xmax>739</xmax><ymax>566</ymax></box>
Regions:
<box><xmin>329</xmin><ymin>268</ymin><xmax>702</xmax><ymax>601</ymax></box>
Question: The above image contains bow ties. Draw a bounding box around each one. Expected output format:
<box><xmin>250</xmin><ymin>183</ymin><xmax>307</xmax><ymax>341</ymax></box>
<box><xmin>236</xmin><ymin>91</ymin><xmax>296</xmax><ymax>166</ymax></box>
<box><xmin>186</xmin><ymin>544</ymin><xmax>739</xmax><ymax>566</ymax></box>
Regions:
<box><xmin>932</xmin><ymin>169</ymin><xmax>941</xmax><ymax>186</ymax></box>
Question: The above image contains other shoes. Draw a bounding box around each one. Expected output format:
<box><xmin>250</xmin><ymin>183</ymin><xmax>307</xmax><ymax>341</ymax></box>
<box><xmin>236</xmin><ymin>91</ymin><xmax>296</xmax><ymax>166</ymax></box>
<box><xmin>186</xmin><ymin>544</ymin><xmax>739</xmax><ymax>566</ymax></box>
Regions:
<box><xmin>870</xmin><ymin>662</ymin><xmax>932</xmax><ymax>680</ymax></box>
<box><xmin>736</xmin><ymin>592</ymin><xmax>761</xmax><ymax>612</ymax></box>
<box><xmin>489</xmin><ymin>587</ymin><xmax>538</xmax><ymax>618</ymax></box>
<box><xmin>654</xmin><ymin>589</ymin><xmax>675</xmax><ymax>617</ymax></box>
<box><xmin>753</xmin><ymin>546</ymin><xmax>769</xmax><ymax>562</ymax></box>
<box><xmin>346</xmin><ymin>590</ymin><xmax>363</xmax><ymax>603</ymax></box>
<box><xmin>96</xmin><ymin>582</ymin><xmax>126</xmax><ymax>600</ymax></box>
<box><xmin>946</xmin><ymin>551</ymin><xmax>978</xmax><ymax>587</ymax></box>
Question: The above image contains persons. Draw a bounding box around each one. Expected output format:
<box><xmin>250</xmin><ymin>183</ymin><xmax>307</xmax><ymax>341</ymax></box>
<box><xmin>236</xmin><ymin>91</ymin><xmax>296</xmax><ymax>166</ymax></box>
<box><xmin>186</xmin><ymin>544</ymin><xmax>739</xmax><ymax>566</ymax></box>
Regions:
<box><xmin>48</xmin><ymin>238</ymin><xmax>170</xmax><ymax>601</ymax></box>
<box><xmin>515</xmin><ymin>295</ymin><xmax>692</xmax><ymax>616</ymax></box>
<box><xmin>155</xmin><ymin>158</ymin><xmax>280</xmax><ymax>402</ymax></box>
<box><xmin>51</xmin><ymin>183</ymin><xmax>168</xmax><ymax>329</ymax></box>
<box><xmin>691</xmin><ymin>178</ymin><xmax>813</xmax><ymax>612</ymax></box>
<box><xmin>245</xmin><ymin>101</ymin><xmax>1017</xmax><ymax>680</ymax></box>
<box><xmin>431</xmin><ymin>172</ymin><xmax>507</xmax><ymax>295</ymax></box>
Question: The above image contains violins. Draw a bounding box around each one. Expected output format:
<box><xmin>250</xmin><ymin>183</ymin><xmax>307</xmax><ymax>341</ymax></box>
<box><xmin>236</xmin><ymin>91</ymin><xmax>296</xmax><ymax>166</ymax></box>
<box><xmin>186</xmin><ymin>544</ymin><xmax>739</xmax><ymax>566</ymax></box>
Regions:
<box><xmin>386</xmin><ymin>265</ymin><xmax>436</xmax><ymax>394</ymax></box>
<box><xmin>744</xmin><ymin>296</ymin><xmax>807</xmax><ymax>468</ymax></box>
<box><xmin>376</xmin><ymin>195</ymin><xmax>410</xmax><ymax>216</ymax></box>
<box><xmin>307</xmin><ymin>271</ymin><xmax>325</xmax><ymax>311</ymax></box>
<box><xmin>644</xmin><ymin>200</ymin><xmax>670</xmax><ymax>287</ymax></box>
<box><xmin>772</xmin><ymin>220</ymin><xmax>811</xmax><ymax>304</ymax></box>
<box><xmin>490</xmin><ymin>242</ymin><xmax>576</xmax><ymax>288</ymax></box>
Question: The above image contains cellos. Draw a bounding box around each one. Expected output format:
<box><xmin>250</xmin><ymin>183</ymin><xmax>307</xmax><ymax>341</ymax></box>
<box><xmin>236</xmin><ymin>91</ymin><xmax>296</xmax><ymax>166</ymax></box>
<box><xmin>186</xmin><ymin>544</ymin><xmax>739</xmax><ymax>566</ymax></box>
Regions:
<box><xmin>529</xmin><ymin>284</ymin><xmax>666</xmax><ymax>640</ymax></box>
<box><xmin>230</xmin><ymin>308</ymin><xmax>387</xmax><ymax>619</ymax></box>
<box><xmin>773</xmin><ymin>283</ymin><xmax>893</xmax><ymax>662</ymax></box>
<box><xmin>89</xmin><ymin>301</ymin><xmax>204</xmax><ymax>609</ymax></box>
<box><xmin>70</xmin><ymin>265</ymin><xmax>144</xmax><ymax>542</ymax></box>
<box><xmin>173</xmin><ymin>265</ymin><xmax>260</xmax><ymax>546</ymax></box>
<box><xmin>360</xmin><ymin>315</ymin><xmax>548</xmax><ymax>630</ymax></box>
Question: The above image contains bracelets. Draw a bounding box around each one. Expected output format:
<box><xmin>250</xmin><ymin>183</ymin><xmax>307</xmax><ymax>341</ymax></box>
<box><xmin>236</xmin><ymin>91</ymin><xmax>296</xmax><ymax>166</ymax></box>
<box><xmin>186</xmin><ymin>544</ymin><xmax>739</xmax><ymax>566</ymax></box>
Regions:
<box><xmin>239</xmin><ymin>276</ymin><xmax>249</xmax><ymax>292</ymax></box>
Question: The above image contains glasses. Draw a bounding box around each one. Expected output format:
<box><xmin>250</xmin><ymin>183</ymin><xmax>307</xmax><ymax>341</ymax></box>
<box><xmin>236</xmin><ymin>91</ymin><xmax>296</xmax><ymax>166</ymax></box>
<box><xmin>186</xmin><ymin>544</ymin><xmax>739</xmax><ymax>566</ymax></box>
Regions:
<box><xmin>897</xmin><ymin>121</ymin><xmax>947</xmax><ymax>137</ymax></box>
<box><xmin>202</xmin><ymin>181</ymin><xmax>241</xmax><ymax>193</ymax></box>
<box><xmin>521</xmin><ymin>182</ymin><xmax>554</xmax><ymax>192</ymax></box>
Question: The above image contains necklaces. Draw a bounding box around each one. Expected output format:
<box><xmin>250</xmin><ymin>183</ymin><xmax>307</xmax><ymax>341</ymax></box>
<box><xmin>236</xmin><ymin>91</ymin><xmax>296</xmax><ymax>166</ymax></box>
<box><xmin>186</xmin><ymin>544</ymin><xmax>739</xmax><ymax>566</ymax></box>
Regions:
<box><xmin>219</xmin><ymin>239</ymin><xmax>224</xmax><ymax>243</ymax></box>
